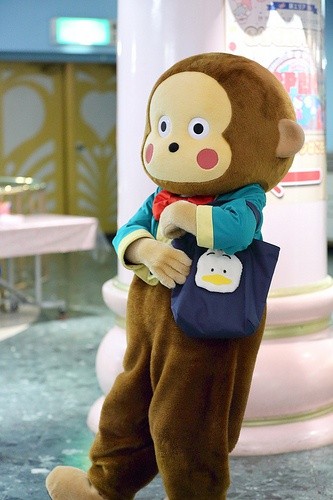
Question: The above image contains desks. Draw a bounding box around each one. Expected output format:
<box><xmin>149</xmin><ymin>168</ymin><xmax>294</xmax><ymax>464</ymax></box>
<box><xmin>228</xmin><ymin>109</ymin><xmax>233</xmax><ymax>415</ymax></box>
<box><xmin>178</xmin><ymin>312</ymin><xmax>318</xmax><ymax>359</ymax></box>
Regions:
<box><xmin>0</xmin><ymin>215</ymin><xmax>107</xmax><ymax>319</ymax></box>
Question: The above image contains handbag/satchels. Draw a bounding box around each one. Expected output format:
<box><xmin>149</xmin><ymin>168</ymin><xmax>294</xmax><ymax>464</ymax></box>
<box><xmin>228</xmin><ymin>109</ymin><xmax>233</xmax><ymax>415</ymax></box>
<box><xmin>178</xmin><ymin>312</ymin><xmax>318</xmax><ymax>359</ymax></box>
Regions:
<box><xmin>168</xmin><ymin>199</ymin><xmax>280</xmax><ymax>340</ymax></box>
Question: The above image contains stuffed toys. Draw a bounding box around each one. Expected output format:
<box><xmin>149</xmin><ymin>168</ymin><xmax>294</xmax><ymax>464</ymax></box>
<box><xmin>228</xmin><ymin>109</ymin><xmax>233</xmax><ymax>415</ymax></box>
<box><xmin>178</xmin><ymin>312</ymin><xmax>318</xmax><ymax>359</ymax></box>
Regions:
<box><xmin>45</xmin><ymin>52</ymin><xmax>305</xmax><ymax>500</ymax></box>
